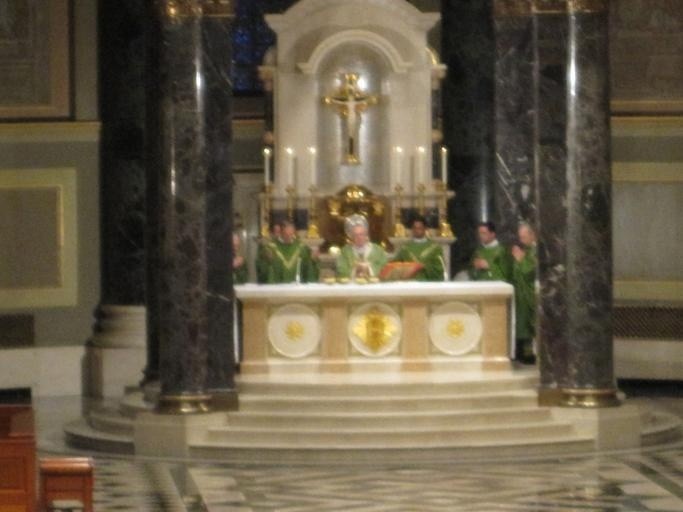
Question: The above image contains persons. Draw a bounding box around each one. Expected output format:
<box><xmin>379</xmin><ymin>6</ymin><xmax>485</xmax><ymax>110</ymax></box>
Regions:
<box><xmin>329</xmin><ymin>90</ymin><xmax>371</xmax><ymax>144</ymax></box>
<box><xmin>331</xmin><ymin>212</ymin><xmax>390</xmax><ymax>283</ymax></box>
<box><xmin>231</xmin><ymin>232</ymin><xmax>251</xmax><ymax>370</ymax></box>
<box><xmin>390</xmin><ymin>215</ymin><xmax>447</xmax><ymax>282</ymax></box>
<box><xmin>509</xmin><ymin>217</ymin><xmax>537</xmax><ymax>366</ymax></box>
<box><xmin>468</xmin><ymin>219</ymin><xmax>511</xmax><ymax>281</ymax></box>
<box><xmin>255</xmin><ymin>219</ymin><xmax>282</xmax><ymax>264</ymax></box>
<box><xmin>254</xmin><ymin>217</ymin><xmax>321</xmax><ymax>286</ymax></box>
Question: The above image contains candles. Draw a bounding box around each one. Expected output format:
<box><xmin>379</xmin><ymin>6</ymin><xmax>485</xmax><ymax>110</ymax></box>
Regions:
<box><xmin>264</xmin><ymin>147</ymin><xmax>317</xmax><ymax>184</ymax></box>
<box><xmin>397</xmin><ymin>148</ymin><xmax>448</xmax><ymax>184</ymax></box>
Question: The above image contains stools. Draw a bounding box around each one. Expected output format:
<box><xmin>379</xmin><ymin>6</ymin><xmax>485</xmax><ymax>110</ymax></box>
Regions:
<box><xmin>40</xmin><ymin>458</ymin><xmax>93</xmax><ymax>512</ymax></box>
